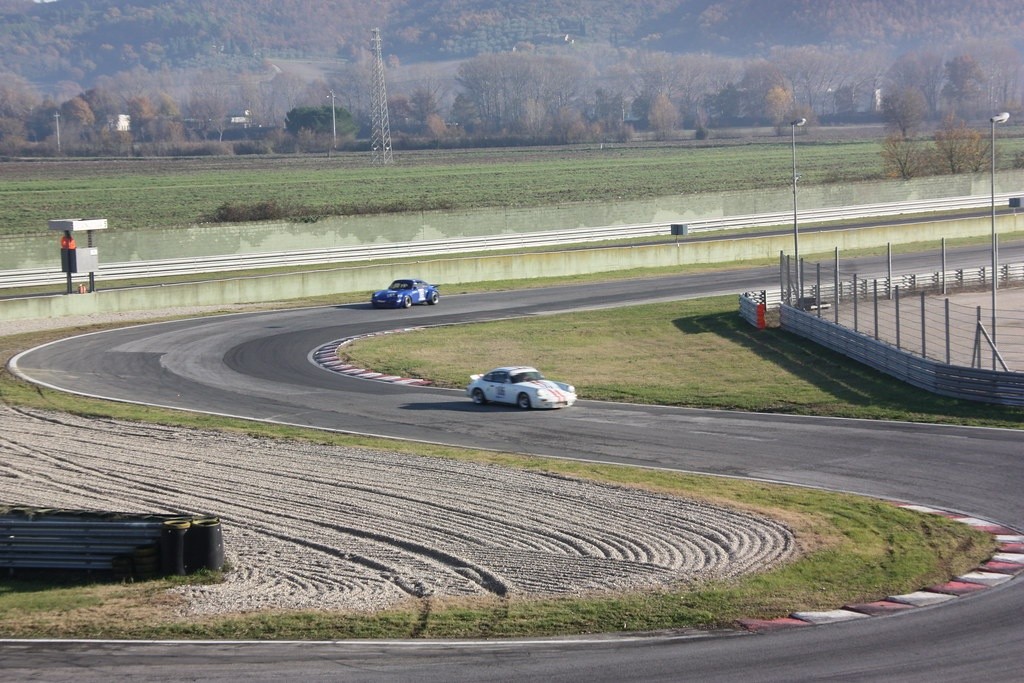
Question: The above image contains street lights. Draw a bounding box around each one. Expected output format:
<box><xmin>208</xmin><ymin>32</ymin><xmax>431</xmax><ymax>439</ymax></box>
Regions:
<box><xmin>989</xmin><ymin>111</ymin><xmax>1010</xmax><ymax>371</ymax></box>
<box><xmin>789</xmin><ymin>118</ymin><xmax>807</xmax><ymax>311</ymax></box>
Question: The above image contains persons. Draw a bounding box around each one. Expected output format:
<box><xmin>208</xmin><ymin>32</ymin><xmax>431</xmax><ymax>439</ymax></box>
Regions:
<box><xmin>60</xmin><ymin>229</ymin><xmax>76</xmax><ymax>252</ymax></box>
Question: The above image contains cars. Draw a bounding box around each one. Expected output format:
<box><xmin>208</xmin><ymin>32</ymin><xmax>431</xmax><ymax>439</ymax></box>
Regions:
<box><xmin>371</xmin><ymin>279</ymin><xmax>439</xmax><ymax>308</ymax></box>
<box><xmin>464</xmin><ymin>366</ymin><xmax>577</xmax><ymax>410</ymax></box>
